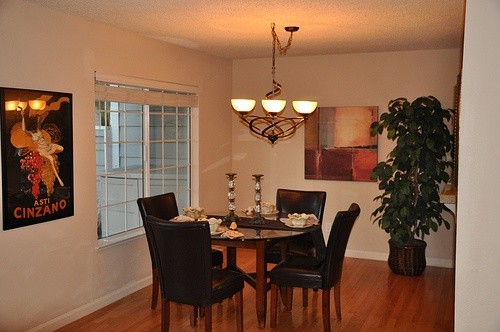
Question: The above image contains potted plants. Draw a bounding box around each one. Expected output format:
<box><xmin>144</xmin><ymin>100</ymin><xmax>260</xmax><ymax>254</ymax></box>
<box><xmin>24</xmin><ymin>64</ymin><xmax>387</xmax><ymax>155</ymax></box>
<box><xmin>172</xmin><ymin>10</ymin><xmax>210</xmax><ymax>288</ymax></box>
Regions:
<box><xmin>368</xmin><ymin>91</ymin><xmax>456</xmax><ymax>278</ymax></box>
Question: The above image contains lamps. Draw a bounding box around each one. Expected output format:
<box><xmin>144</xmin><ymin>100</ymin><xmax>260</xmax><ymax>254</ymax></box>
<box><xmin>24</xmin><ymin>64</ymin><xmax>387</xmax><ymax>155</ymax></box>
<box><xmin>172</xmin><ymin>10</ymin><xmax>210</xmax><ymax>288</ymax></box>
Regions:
<box><xmin>230</xmin><ymin>22</ymin><xmax>319</xmax><ymax>146</ymax></box>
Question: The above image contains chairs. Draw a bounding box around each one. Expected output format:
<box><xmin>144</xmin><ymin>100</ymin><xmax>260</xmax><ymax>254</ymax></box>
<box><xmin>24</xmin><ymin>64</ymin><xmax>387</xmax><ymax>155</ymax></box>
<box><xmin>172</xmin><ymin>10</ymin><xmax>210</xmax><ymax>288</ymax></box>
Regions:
<box><xmin>136</xmin><ymin>190</ymin><xmax>224</xmax><ymax>319</ymax></box>
<box><xmin>144</xmin><ymin>214</ymin><xmax>244</xmax><ymax>332</ymax></box>
<box><xmin>266</xmin><ymin>187</ymin><xmax>327</xmax><ymax>309</ymax></box>
<box><xmin>269</xmin><ymin>202</ymin><xmax>362</xmax><ymax>332</ymax></box>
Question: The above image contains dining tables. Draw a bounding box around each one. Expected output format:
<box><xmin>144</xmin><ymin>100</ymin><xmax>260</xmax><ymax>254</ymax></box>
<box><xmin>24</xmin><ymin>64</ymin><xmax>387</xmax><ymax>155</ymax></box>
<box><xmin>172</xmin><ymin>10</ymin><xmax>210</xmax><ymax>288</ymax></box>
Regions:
<box><xmin>206</xmin><ymin>209</ymin><xmax>322</xmax><ymax>329</ymax></box>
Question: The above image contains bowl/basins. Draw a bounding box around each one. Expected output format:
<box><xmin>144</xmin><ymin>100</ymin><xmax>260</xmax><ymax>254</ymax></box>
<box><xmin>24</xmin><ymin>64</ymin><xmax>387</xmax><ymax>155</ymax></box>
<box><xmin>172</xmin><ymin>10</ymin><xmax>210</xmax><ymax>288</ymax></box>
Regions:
<box><xmin>261</xmin><ymin>202</ymin><xmax>276</xmax><ymax>213</ymax></box>
<box><xmin>183</xmin><ymin>208</ymin><xmax>204</xmax><ymax>219</ymax></box>
<box><xmin>201</xmin><ymin>218</ymin><xmax>222</xmax><ymax>232</ymax></box>
<box><xmin>287</xmin><ymin>212</ymin><xmax>315</xmax><ymax>226</ymax></box>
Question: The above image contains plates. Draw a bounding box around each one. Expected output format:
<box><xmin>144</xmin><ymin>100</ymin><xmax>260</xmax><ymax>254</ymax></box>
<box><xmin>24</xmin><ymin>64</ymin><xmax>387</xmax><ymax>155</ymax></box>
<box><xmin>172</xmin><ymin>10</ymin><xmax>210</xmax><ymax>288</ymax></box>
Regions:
<box><xmin>260</xmin><ymin>210</ymin><xmax>279</xmax><ymax>215</ymax></box>
<box><xmin>210</xmin><ymin>228</ymin><xmax>224</xmax><ymax>235</ymax></box>
<box><xmin>280</xmin><ymin>218</ymin><xmax>319</xmax><ymax>228</ymax></box>
